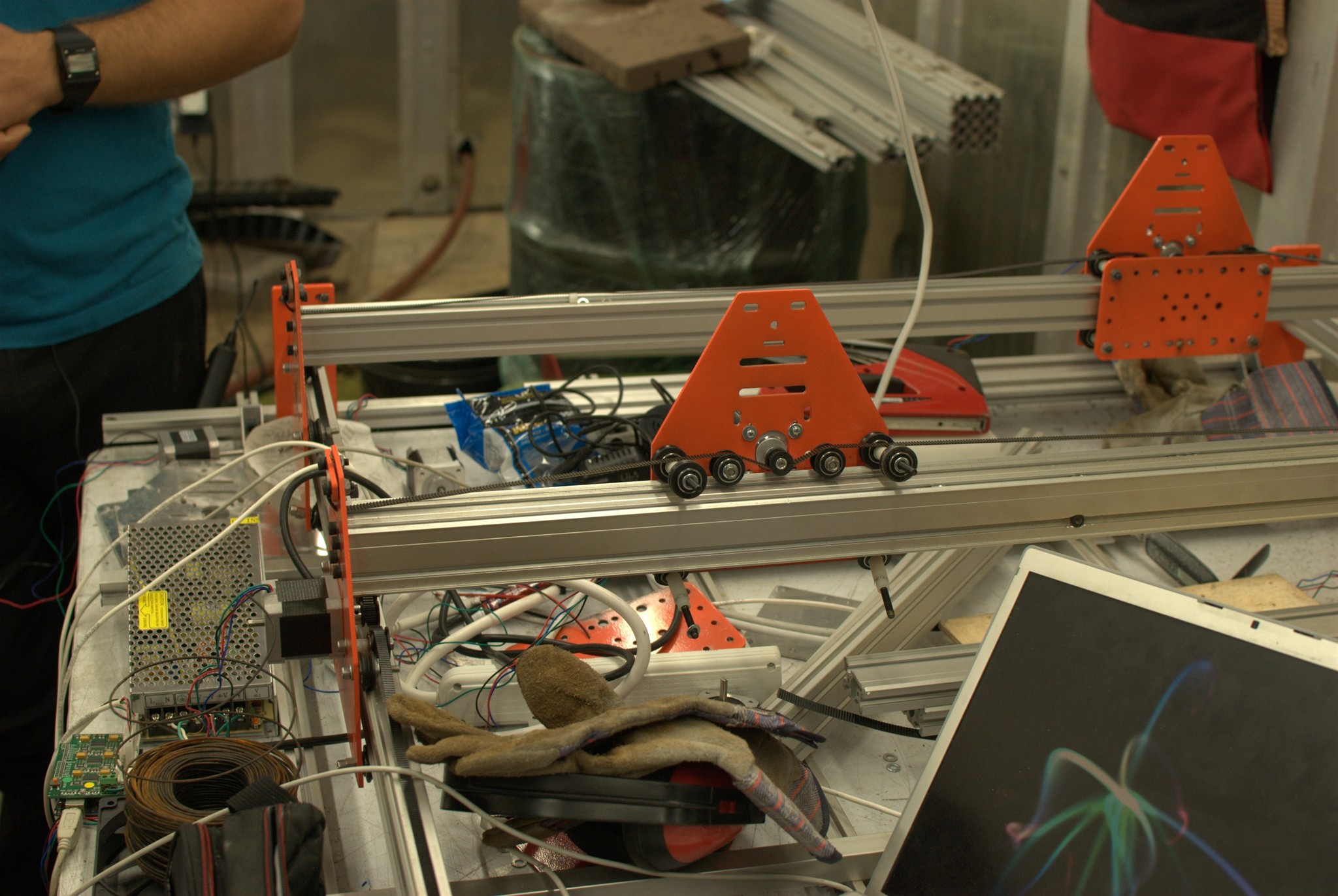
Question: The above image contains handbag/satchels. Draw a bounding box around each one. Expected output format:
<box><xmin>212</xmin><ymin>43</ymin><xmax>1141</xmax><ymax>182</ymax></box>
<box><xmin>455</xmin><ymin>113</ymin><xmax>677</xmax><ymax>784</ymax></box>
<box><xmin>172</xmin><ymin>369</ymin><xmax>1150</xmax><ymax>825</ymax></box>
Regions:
<box><xmin>1090</xmin><ymin>0</ymin><xmax>1288</xmax><ymax>193</ymax></box>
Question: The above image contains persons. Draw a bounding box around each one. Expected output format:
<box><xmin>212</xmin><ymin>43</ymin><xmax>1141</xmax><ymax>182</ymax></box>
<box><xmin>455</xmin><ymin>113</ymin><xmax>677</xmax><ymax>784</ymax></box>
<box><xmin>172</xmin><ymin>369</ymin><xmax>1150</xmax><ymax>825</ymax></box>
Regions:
<box><xmin>0</xmin><ymin>0</ymin><xmax>304</xmax><ymax>896</ymax></box>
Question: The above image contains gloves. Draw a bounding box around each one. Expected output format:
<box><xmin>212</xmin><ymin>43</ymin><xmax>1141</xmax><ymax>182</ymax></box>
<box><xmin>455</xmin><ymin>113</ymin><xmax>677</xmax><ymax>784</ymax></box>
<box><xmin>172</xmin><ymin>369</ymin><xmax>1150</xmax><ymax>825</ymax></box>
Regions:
<box><xmin>387</xmin><ymin>643</ymin><xmax>846</xmax><ymax>865</ymax></box>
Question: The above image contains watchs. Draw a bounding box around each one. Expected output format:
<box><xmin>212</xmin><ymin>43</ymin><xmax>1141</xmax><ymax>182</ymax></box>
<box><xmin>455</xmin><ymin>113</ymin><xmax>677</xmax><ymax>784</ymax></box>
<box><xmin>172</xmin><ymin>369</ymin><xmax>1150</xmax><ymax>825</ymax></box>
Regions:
<box><xmin>47</xmin><ymin>24</ymin><xmax>100</xmax><ymax>112</ymax></box>
